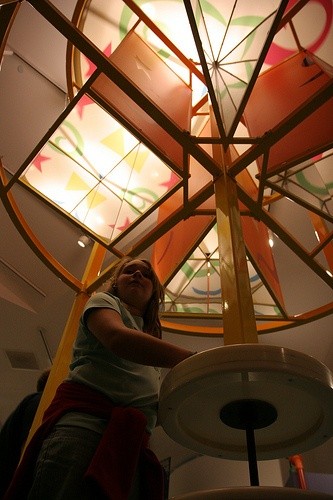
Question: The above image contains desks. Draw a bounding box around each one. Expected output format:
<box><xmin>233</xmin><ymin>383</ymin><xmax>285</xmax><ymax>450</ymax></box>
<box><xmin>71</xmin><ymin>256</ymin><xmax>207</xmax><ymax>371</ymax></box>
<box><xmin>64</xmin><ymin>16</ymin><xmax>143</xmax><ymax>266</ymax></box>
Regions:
<box><xmin>159</xmin><ymin>343</ymin><xmax>332</xmax><ymax>500</ymax></box>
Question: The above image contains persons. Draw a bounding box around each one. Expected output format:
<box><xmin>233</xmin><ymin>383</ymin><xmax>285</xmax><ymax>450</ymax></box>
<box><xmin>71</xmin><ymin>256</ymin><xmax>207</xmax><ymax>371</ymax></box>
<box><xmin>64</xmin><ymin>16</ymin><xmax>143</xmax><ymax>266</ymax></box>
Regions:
<box><xmin>0</xmin><ymin>255</ymin><xmax>198</xmax><ymax>500</ymax></box>
<box><xmin>0</xmin><ymin>369</ymin><xmax>51</xmax><ymax>499</ymax></box>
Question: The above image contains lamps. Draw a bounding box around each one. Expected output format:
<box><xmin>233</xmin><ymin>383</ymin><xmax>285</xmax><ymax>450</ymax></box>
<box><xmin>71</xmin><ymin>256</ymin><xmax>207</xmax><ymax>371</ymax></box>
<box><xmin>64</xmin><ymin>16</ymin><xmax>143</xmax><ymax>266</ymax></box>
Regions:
<box><xmin>77</xmin><ymin>234</ymin><xmax>92</xmax><ymax>248</ymax></box>
